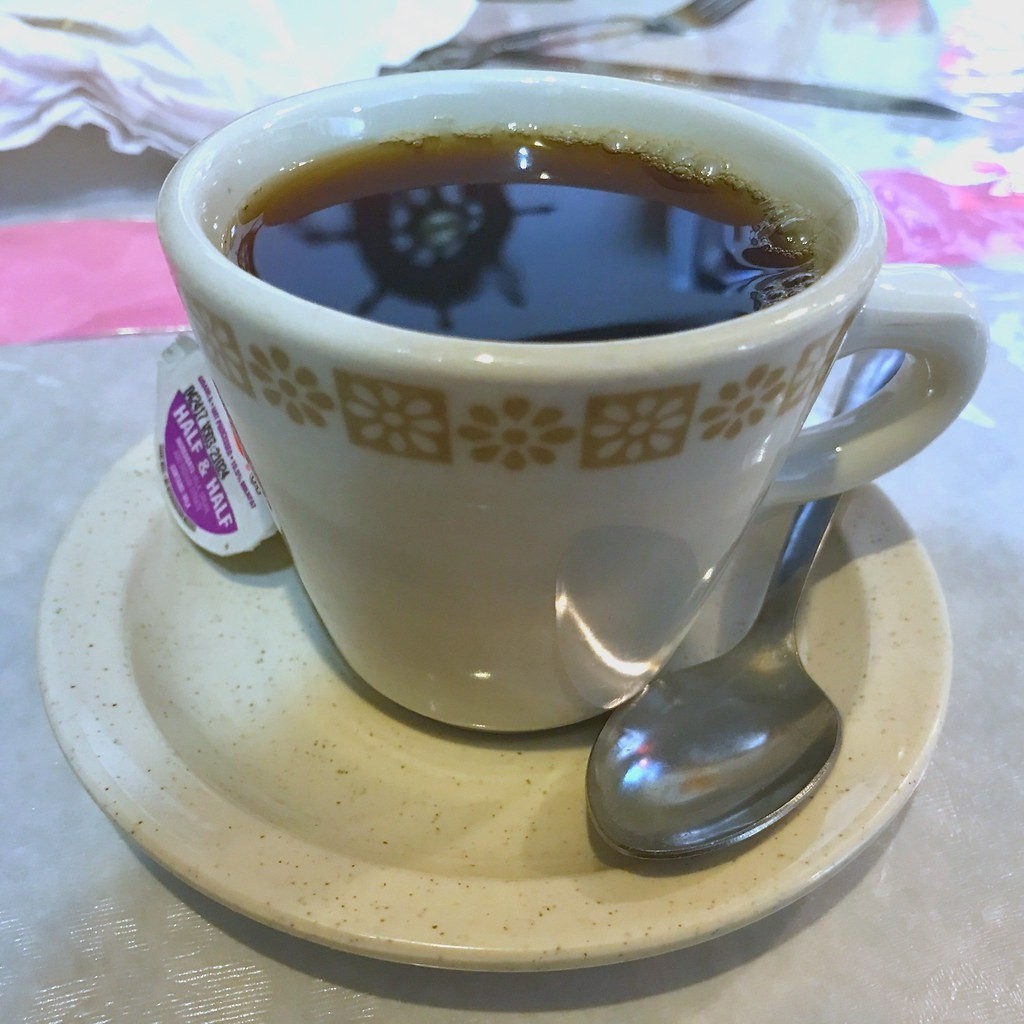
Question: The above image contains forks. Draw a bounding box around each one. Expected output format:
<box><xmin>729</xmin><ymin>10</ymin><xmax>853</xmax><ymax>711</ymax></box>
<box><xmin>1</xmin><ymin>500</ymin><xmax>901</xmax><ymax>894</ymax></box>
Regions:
<box><xmin>394</xmin><ymin>0</ymin><xmax>746</xmax><ymax>73</ymax></box>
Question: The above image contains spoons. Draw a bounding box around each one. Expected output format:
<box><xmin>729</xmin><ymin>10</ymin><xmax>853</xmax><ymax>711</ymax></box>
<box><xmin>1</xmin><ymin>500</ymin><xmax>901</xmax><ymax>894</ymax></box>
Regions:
<box><xmin>585</xmin><ymin>347</ymin><xmax>906</xmax><ymax>860</ymax></box>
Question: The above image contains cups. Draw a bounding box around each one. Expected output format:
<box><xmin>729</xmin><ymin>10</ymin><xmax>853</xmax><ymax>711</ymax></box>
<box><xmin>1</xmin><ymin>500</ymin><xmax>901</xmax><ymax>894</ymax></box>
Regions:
<box><xmin>153</xmin><ymin>70</ymin><xmax>989</xmax><ymax>732</ymax></box>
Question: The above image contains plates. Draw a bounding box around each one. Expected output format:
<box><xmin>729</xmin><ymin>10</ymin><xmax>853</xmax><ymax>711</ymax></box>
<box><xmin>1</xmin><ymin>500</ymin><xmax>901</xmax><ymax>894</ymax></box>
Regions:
<box><xmin>35</xmin><ymin>428</ymin><xmax>958</xmax><ymax>971</ymax></box>
